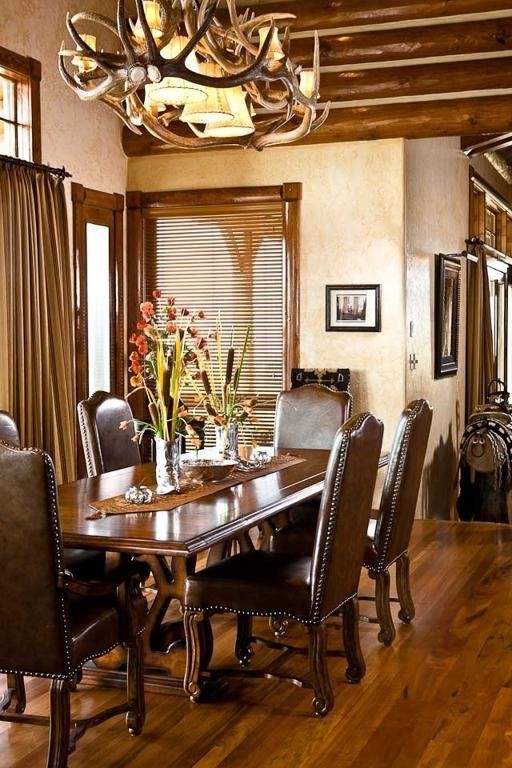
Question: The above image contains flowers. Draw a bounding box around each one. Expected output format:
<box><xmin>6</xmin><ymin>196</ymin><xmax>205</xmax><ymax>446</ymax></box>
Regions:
<box><xmin>202</xmin><ymin>312</ymin><xmax>277</xmax><ymax>457</ymax></box>
<box><xmin>120</xmin><ymin>288</ymin><xmax>213</xmax><ymax>446</ymax></box>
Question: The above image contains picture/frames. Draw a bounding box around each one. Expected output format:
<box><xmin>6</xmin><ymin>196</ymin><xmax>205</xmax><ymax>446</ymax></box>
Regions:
<box><xmin>430</xmin><ymin>252</ymin><xmax>463</xmax><ymax>380</ymax></box>
<box><xmin>152</xmin><ymin>430</ymin><xmax>180</xmax><ymax>495</ymax></box>
<box><xmin>323</xmin><ymin>281</ymin><xmax>384</xmax><ymax>335</ymax></box>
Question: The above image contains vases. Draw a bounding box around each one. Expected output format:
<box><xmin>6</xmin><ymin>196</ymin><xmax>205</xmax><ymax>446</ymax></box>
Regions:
<box><xmin>216</xmin><ymin>422</ymin><xmax>241</xmax><ymax>463</ymax></box>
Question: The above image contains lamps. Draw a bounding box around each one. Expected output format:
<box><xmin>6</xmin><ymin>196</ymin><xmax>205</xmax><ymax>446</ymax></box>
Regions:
<box><xmin>57</xmin><ymin>0</ymin><xmax>336</xmax><ymax>153</ymax></box>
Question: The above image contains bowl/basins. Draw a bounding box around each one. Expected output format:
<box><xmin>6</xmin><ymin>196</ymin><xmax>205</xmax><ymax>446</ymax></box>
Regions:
<box><xmin>180</xmin><ymin>459</ymin><xmax>239</xmax><ymax>479</ymax></box>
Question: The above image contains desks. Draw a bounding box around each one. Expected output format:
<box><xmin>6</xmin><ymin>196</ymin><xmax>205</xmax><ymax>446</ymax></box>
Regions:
<box><xmin>48</xmin><ymin>443</ymin><xmax>395</xmax><ymax>700</ymax></box>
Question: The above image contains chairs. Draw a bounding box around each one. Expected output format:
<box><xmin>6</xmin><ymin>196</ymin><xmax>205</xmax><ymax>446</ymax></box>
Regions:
<box><xmin>77</xmin><ymin>389</ymin><xmax>151</xmax><ymax>611</ymax></box>
<box><xmin>0</xmin><ymin>410</ymin><xmax>23</xmax><ymax>445</ymax></box>
<box><xmin>0</xmin><ymin>439</ymin><xmax>154</xmax><ymax>767</ymax></box>
<box><xmin>272</xmin><ymin>382</ymin><xmax>355</xmax><ymax>523</ymax></box>
<box><xmin>267</xmin><ymin>398</ymin><xmax>438</xmax><ymax>646</ymax></box>
<box><xmin>180</xmin><ymin>410</ymin><xmax>388</xmax><ymax>721</ymax></box>
